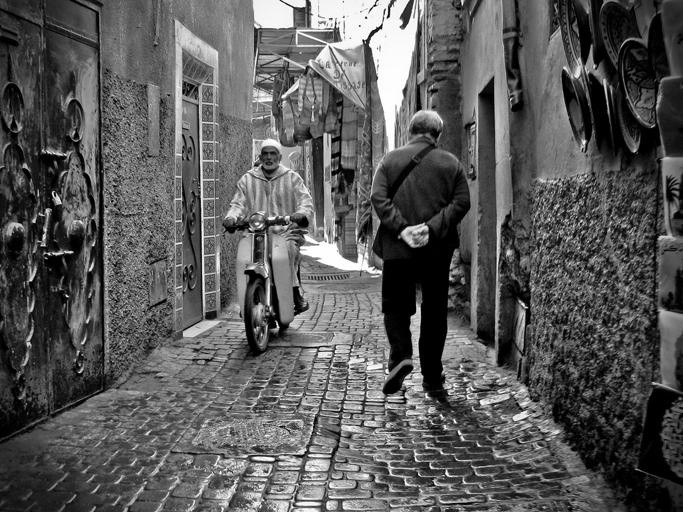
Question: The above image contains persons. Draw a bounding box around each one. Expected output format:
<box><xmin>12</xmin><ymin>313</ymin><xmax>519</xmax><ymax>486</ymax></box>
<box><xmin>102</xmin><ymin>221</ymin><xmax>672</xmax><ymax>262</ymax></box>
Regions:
<box><xmin>371</xmin><ymin>111</ymin><xmax>470</xmax><ymax>394</ymax></box>
<box><xmin>222</xmin><ymin>139</ymin><xmax>314</xmax><ymax>312</ymax></box>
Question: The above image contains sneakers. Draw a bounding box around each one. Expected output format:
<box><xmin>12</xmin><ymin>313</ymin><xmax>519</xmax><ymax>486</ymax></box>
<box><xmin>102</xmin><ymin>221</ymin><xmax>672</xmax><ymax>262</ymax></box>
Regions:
<box><xmin>382</xmin><ymin>359</ymin><xmax>413</xmax><ymax>395</ymax></box>
<box><xmin>422</xmin><ymin>376</ymin><xmax>445</xmax><ymax>393</ymax></box>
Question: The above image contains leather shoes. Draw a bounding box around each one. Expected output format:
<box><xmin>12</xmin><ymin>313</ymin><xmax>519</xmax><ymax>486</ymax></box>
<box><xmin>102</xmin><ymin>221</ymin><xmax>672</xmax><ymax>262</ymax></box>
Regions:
<box><xmin>293</xmin><ymin>296</ymin><xmax>310</xmax><ymax>313</ymax></box>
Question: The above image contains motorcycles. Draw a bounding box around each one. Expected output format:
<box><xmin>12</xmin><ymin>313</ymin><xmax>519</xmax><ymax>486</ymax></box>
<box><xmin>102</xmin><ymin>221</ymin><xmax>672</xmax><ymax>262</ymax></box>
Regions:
<box><xmin>220</xmin><ymin>212</ymin><xmax>303</xmax><ymax>355</ymax></box>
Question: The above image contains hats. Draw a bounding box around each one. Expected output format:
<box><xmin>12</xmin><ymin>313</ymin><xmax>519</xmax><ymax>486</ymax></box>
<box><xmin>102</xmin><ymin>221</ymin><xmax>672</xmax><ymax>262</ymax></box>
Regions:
<box><xmin>260</xmin><ymin>138</ymin><xmax>282</xmax><ymax>155</ymax></box>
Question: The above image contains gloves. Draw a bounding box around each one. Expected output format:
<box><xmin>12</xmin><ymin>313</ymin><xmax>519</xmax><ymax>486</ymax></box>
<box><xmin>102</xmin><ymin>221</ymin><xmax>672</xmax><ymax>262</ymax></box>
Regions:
<box><xmin>289</xmin><ymin>212</ymin><xmax>309</xmax><ymax>228</ymax></box>
<box><xmin>221</xmin><ymin>215</ymin><xmax>237</xmax><ymax>234</ymax></box>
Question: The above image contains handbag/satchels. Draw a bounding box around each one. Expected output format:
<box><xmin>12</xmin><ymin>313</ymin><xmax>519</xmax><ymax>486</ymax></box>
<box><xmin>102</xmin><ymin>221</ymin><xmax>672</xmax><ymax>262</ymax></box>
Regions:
<box><xmin>371</xmin><ymin>223</ymin><xmax>383</xmax><ymax>260</ymax></box>
